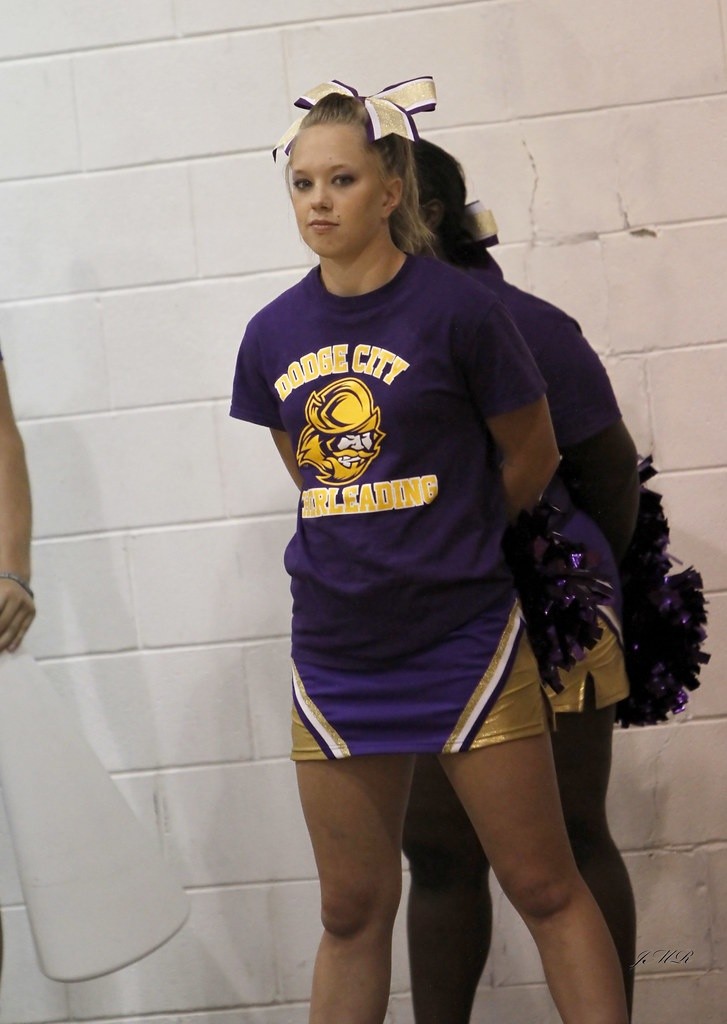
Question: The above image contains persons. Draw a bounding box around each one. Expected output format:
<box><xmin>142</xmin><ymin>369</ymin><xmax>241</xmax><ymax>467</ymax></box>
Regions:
<box><xmin>230</xmin><ymin>71</ymin><xmax>631</xmax><ymax>1024</ymax></box>
<box><xmin>0</xmin><ymin>350</ymin><xmax>36</xmax><ymax>658</ymax></box>
<box><xmin>403</xmin><ymin>135</ymin><xmax>636</xmax><ymax>1024</ymax></box>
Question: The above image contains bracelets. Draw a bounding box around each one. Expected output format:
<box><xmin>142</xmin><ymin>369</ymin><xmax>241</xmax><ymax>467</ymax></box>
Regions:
<box><xmin>0</xmin><ymin>574</ymin><xmax>34</xmax><ymax>598</ymax></box>
